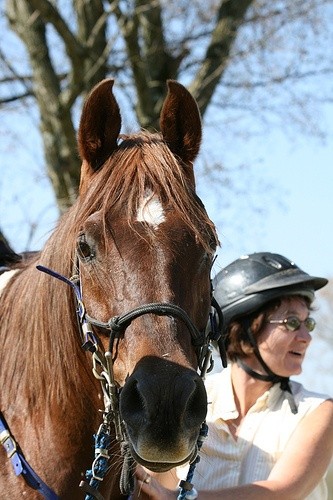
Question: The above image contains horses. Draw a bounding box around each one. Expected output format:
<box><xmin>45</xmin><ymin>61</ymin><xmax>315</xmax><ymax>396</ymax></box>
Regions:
<box><xmin>0</xmin><ymin>78</ymin><xmax>222</xmax><ymax>500</ymax></box>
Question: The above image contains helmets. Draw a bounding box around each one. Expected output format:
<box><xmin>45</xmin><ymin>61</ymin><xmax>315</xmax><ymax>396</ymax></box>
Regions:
<box><xmin>207</xmin><ymin>252</ymin><xmax>329</xmax><ymax>339</ymax></box>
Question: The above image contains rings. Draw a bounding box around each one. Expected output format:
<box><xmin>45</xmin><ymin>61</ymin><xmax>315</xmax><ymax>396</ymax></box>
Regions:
<box><xmin>143</xmin><ymin>473</ymin><xmax>152</xmax><ymax>484</ymax></box>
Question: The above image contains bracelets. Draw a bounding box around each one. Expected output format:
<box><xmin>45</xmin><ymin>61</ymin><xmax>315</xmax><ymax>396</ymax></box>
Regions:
<box><xmin>176</xmin><ymin>487</ymin><xmax>198</xmax><ymax>500</ymax></box>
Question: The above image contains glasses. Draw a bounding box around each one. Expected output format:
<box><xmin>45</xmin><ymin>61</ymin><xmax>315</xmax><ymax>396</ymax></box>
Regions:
<box><xmin>263</xmin><ymin>317</ymin><xmax>316</xmax><ymax>330</ymax></box>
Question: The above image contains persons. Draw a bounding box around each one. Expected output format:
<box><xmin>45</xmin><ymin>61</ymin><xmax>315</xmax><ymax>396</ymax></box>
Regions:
<box><xmin>129</xmin><ymin>252</ymin><xmax>333</xmax><ymax>500</ymax></box>
